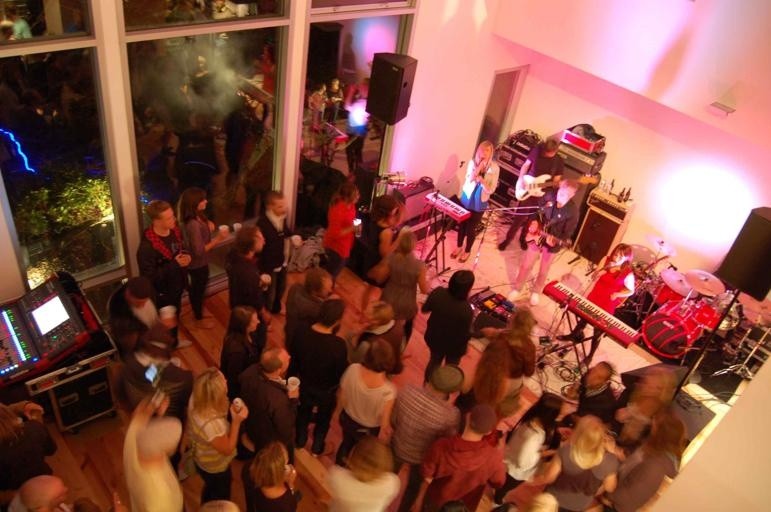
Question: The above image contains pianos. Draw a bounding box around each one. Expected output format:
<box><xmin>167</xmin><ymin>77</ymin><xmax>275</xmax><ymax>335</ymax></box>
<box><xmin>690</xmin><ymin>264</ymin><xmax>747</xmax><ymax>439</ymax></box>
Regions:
<box><xmin>424</xmin><ymin>192</ymin><xmax>471</xmax><ymax>224</ymax></box>
<box><xmin>543</xmin><ymin>280</ymin><xmax>640</xmax><ymax>349</ymax></box>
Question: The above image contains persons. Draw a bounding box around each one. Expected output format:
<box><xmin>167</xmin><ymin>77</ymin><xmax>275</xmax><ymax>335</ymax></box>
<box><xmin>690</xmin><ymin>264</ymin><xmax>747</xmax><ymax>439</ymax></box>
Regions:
<box><xmin>0</xmin><ymin>173</ymin><xmax>689</xmax><ymax>511</ymax></box>
<box><xmin>448</xmin><ymin>140</ymin><xmax>500</xmax><ymax>262</ymax></box>
<box><xmin>133</xmin><ymin>1</ymin><xmax>369</xmax><ymax>238</ymax></box>
<box><xmin>500</xmin><ymin>136</ymin><xmax>564</xmax><ymax>252</ymax></box>
<box><xmin>3</xmin><ymin>8</ymin><xmax>32</xmax><ymax>39</ymax></box>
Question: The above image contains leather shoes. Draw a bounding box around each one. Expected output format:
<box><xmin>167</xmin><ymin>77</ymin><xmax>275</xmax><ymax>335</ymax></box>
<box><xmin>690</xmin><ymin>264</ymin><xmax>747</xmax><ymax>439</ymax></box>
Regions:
<box><xmin>458</xmin><ymin>253</ymin><xmax>471</xmax><ymax>262</ymax></box>
<box><xmin>450</xmin><ymin>248</ymin><xmax>463</xmax><ymax>259</ymax></box>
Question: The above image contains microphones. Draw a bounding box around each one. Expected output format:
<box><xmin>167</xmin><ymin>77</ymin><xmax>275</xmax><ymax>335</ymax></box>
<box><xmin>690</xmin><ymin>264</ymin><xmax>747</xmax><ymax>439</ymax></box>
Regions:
<box><xmin>600</xmin><ymin>262</ymin><xmax>618</xmax><ymax>271</ymax></box>
<box><xmin>540</xmin><ymin>201</ymin><xmax>554</xmax><ymax>213</ymax></box>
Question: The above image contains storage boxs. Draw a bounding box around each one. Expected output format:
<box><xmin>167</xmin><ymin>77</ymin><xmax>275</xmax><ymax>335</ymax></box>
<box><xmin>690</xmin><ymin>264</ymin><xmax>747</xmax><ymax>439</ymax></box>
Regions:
<box><xmin>488</xmin><ymin>144</ymin><xmax>532</xmax><ymax>212</ymax></box>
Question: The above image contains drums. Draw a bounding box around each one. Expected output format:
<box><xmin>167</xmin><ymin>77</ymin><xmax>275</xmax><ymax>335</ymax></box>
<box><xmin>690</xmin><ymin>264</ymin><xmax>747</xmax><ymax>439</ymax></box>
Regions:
<box><xmin>718</xmin><ymin>305</ymin><xmax>742</xmax><ymax>330</ymax></box>
<box><xmin>642</xmin><ymin>299</ymin><xmax>701</xmax><ymax>358</ymax></box>
<box><xmin>689</xmin><ymin>290</ymin><xmax>734</xmax><ymax>332</ymax></box>
<box><xmin>649</xmin><ymin>277</ymin><xmax>684</xmax><ymax>308</ymax></box>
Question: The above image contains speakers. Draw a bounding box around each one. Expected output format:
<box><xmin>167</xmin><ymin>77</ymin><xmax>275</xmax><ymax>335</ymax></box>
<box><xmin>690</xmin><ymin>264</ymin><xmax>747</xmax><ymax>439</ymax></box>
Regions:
<box><xmin>717</xmin><ymin>206</ymin><xmax>771</xmax><ymax>302</ymax></box>
<box><xmin>366</xmin><ymin>51</ymin><xmax>418</xmax><ymax>126</ymax></box>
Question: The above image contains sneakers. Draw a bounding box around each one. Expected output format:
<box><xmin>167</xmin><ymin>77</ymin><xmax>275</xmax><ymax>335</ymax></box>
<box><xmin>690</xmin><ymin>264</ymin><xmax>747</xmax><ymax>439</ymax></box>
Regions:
<box><xmin>530</xmin><ymin>293</ymin><xmax>538</xmax><ymax>306</ymax></box>
<box><xmin>519</xmin><ymin>236</ymin><xmax>528</xmax><ymax>250</ymax></box>
<box><xmin>509</xmin><ymin>289</ymin><xmax>518</xmax><ymax>300</ymax></box>
<box><xmin>498</xmin><ymin>240</ymin><xmax>510</xmax><ymax>250</ymax></box>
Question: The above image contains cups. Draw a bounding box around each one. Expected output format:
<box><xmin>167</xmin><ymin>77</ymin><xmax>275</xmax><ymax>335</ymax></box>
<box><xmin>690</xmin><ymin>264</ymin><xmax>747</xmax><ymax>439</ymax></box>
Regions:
<box><xmin>260</xmin><ymin>274</ymin><xmax>272</xmax><ymax>291</ymax></box>
<box><xmin>291</xmin><ymin>235</ymin><xmax>302</xmax><ymax>246</ymax></box>
<box><xmin>158</xmin><ymin>305</ymin><xmax>178</xmax><ymax>330</ymax></box>
<box><xmin>287</xmin><ymin>376</ymin><xmax>301</xmax><ymax>392</ymax></box>
<box><xmin>31</xmin><ymin>409</ymin><xmax>44</xmax><ymax>425</ymax></box>
<box><xmin>170</xmin><ymin>356</ymin><xmax>181</xmax><ymax>368</ymax></box>
<box><xmin>179</xmin><ymin>250</ymin><xmax>189</xmax><ymax>265</ymax></box>
<box><xmin>233</xmin><ymin>222</ymin><xmax>242</xmax><ymax>233</ymax></box>
<box><xmin>219</xmin><ymin>224</ymin><xmax>228</xmax><ymax>237</ymax></box>
<box><xmin>285</xmin><ymin>464</ymin><xmax>291</xmax><ymax>477</ymax></box>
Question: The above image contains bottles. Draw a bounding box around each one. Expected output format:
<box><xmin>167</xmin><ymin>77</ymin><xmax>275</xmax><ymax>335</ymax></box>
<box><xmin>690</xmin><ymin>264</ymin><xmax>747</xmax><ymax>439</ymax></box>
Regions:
<box><xmin>599</xmin><ymin>178</ymin><xmax>632</xmax><ymax>203</ymax></box>
<box><xmin>232</xmin><ymin>397</ymin><xmax>242</xmax><ymax>414</ymax></box>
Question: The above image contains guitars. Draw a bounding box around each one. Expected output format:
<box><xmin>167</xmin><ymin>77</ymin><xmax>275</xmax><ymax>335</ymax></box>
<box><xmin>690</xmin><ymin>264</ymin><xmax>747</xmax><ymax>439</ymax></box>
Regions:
<box><xmin>515</xmin><ymin>173</ymin><xmax>598</xmax><ymax>201</ymax></box>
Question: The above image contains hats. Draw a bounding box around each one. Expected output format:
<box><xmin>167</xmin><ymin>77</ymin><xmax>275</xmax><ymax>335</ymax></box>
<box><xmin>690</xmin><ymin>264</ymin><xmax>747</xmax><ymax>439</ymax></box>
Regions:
<box><xmin>432</xmin><ymin>365</ymin><xmax>465</xmax><ymax>393</ymax></box>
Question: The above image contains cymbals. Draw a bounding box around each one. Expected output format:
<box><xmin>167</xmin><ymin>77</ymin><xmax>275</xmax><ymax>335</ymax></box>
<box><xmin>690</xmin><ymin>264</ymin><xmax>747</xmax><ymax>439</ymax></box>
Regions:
<box><xmin>685</xmin><ymin>270</ymin><xmax>726</xmax><ymax>296</ymax></box>
<box><xmin>645</xmin><ymin>234</ymin><xmax>677</xmax><ymax>257</ymax></box>
<box><xmin>742</xmin><ymin>299</ymin><xmax>771</xmax><ymax>325</ymax></box>
<box><xmin>661</xmin><ymin>268</ymin><xmax>699</xmax><ymax>298</ymax></box>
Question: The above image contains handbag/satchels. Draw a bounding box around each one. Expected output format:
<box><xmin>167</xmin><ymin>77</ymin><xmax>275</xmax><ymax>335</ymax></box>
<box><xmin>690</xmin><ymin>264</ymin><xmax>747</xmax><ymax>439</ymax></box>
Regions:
<box><xmin>178</xmin><ymin>443</ymin><xmax>195</xmax><ymax>482</ymax></box>
